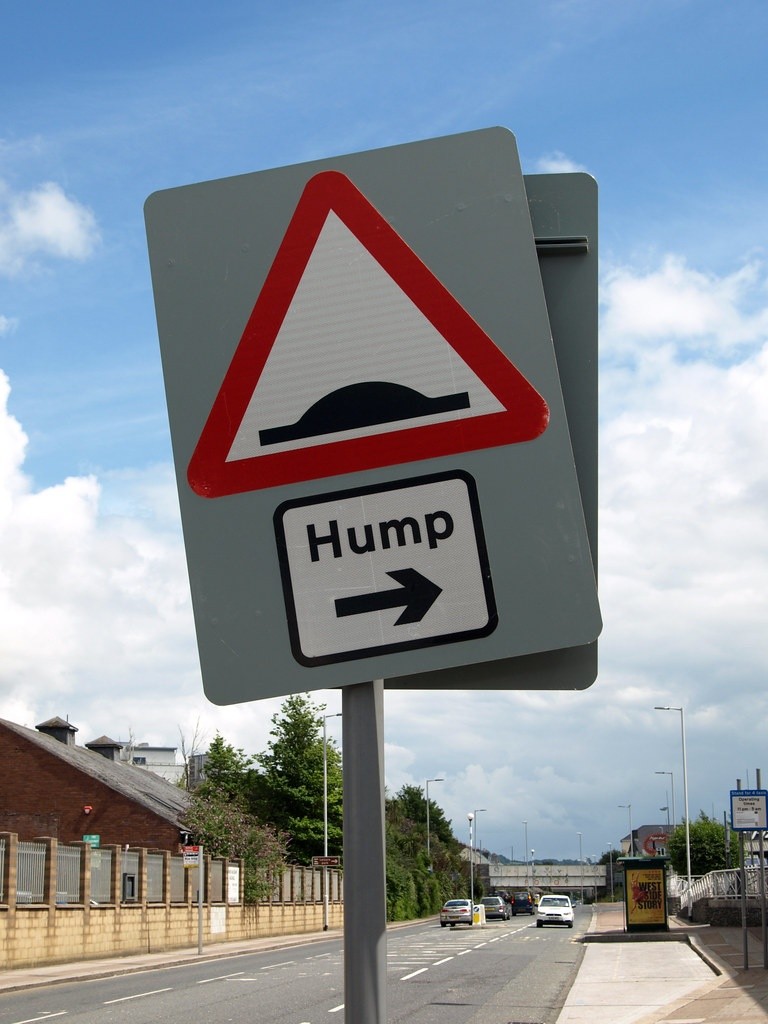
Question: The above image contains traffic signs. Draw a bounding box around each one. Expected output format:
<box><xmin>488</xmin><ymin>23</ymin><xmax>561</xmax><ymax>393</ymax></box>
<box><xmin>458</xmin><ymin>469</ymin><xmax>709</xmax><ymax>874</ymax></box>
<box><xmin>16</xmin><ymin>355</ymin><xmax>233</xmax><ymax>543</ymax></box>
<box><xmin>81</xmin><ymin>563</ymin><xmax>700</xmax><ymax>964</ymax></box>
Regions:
<box><xmin>312</xmin><ymin>856</ymin><xmax>341</xmax><ymax>867</ymax></box>
<box><xmin>143</xmin><ymin>126</ymin><xmax>608</xmax><ymax>710</ymax></box>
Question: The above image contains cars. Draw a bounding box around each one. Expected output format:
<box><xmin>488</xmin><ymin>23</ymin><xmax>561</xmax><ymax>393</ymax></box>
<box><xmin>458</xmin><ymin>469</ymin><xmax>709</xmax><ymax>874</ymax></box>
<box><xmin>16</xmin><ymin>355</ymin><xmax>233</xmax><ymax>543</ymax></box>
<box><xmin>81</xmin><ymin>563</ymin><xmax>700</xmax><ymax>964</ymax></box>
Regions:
<box><xmin>535</xmin><ymin>895</ymin><xmax>576</xmax><ymax>927</ymax></box>
<box><xmin>481</xmin><ymin>897</ymin><xmax>511</xmax><ymax>921</ymax></box>
<box><xmin>570</xmin><ymin>899</ymin><xmax>577</xmax><ymax>908</ymax></box>
<box><xmin>440</xmin><ymin>899</ymin><xmax>477</xmax><ymax>928</ymax></box>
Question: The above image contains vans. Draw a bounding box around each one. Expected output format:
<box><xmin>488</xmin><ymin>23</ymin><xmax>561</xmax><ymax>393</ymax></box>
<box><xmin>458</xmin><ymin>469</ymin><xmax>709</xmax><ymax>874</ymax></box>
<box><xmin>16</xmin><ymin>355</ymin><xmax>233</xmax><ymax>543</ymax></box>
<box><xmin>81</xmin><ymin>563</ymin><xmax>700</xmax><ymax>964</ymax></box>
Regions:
<box><xmin>534</xmin><ymin>894</ymin><xmax>540</xmax><ymax>907</ymax></box>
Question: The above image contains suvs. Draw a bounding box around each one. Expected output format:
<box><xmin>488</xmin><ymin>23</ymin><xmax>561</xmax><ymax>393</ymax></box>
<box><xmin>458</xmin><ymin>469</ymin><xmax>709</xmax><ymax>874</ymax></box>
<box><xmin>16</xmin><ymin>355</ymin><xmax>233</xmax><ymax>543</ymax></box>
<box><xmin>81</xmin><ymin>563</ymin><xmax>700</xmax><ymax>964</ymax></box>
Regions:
<box><xmin>494</xmin><ymin>890</ymin><xmax>512</xmax><ymax>904</ymax></box>
<box><xmin>512</xmin><ymin>891</ymin><xmax>535</xmax><ymax>916</ymax></box>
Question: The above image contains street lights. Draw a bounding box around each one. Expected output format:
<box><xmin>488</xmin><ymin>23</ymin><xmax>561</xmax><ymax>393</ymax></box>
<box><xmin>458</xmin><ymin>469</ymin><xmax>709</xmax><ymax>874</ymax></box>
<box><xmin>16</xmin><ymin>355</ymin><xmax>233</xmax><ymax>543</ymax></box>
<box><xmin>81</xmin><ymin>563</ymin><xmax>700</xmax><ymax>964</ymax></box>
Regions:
<box><xmin>654</xmin><ymin>707</ymin><xmax>695</xmax><ymax>921</ymax></box>
<box><xmin>473</xmin><ymin>808</ymin><xmax>487</xmax><ymax>877</ymax></box>
<box><xmin>531</xmin><ymin>849</ymin><xmax>535</xmax><ymax>892</ymax></box>
<box><xmin>655</xmin><ymin>772</ymin><xmax>676</xmax><ymax>832</ymax></box>
<box><xmin>591</xmin><ymin>855</ymin><xmax>597</xmax><ymax>903</ymax></box>
<box><xmin>323</xmin><ymin>713</ymin><xmax>345</xmax><ymax>929</ymax></box>
<box><xmin>576</xmin><ymin>831</ymin><xmax>584</xmax><ymax>904</ymax></box>
<box><xmin>426</xmin><ymin>778</ymin><xmax>445</xmax><ymax>866</ymax></box>
<box><xmin>618</xmin><ymin>805</ymin><xmax>633</xmax><ymax>858</ymax></box>
<box><xmin>467</xmin><ymin>813</ymin><xmax>475</xmax><ymax>926</ymax></box>
<box><xmin>606</xmin><ymin>842</ymin><xmax>615</xmax><ymax>903</ymax></box>
<box><xmin>522</xmin><ymin>821</ymin><xmax>529</xmax><ymax>893</ymax></box>
